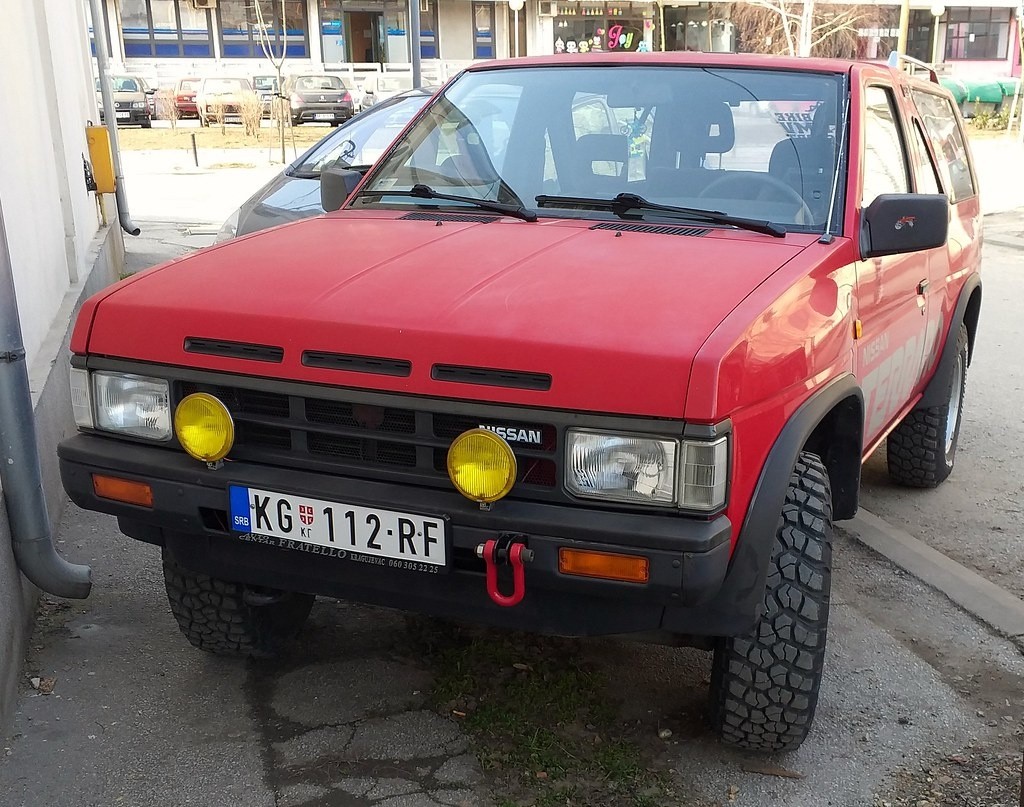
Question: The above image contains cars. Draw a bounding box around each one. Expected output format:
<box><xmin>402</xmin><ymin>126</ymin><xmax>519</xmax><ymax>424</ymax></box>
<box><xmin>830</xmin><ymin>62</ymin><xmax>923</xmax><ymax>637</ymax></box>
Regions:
<box><xmin>92</xmin><ymin>72</ymin><xmax>433</xmax><ymax>129</ymax></box>
<box><xmin>213</xmin><ymin>84</ymin><xmax>628</xmax><ymax>248</ymax></box>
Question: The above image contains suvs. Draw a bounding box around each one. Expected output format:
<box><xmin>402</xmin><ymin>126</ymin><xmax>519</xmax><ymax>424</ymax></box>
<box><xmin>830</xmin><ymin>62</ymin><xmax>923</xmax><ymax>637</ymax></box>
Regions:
<box><xmin>57</xmin><ymin>50</ymin><xmax>984</xmax><ymax>755</ymax></box>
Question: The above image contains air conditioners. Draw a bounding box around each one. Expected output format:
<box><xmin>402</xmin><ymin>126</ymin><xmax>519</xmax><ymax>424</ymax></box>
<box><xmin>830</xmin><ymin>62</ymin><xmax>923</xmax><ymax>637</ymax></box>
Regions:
<box><xmin>538</xmin><ymin>1</ymin><xmax>557</xmax><ymax>17</ymax></box>
<box><xmin>194</xmin><ymin>0</ymin><xmax>216</xmax><ymax>9</ymax></box>
<box><xmin>420</xmin><ymin>0</ymin><xmax>428</xmax><ymax>12</ymax></box>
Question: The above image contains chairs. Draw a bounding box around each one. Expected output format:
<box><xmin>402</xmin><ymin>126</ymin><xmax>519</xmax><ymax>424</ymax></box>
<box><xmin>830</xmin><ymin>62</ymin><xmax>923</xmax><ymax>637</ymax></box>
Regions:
<box><xmin>322</xmin><ymin>82</ymin><xmax>330</xmax><ymax>86</ymax></box>
<box><xmin>758</xmin><ymin>137</ymin><xmax>834</xmax><ymax>204</ymax></box>
<box><xmin>810</xmin><ymin>102</ymin><xmax>836</xmax><ymax>139</ymax></box>
<box><xmin>440</xmin><ymin>116</ymin><xmax>496</xmax><ymax>179</ymax></box>
<box><xmin>558</xmin><ymin>133</ymin><xmax>628</xmax><ymax>196</ymax></box>
<box><xmin>649</xmin><ymin>103</ymin><xmax>736</xmax><ymax>197</ymax></box>
<box><xmin>121</xmin><ymin>81</ymin><xmax>135</xmax><ymax>90</ymax></box>
